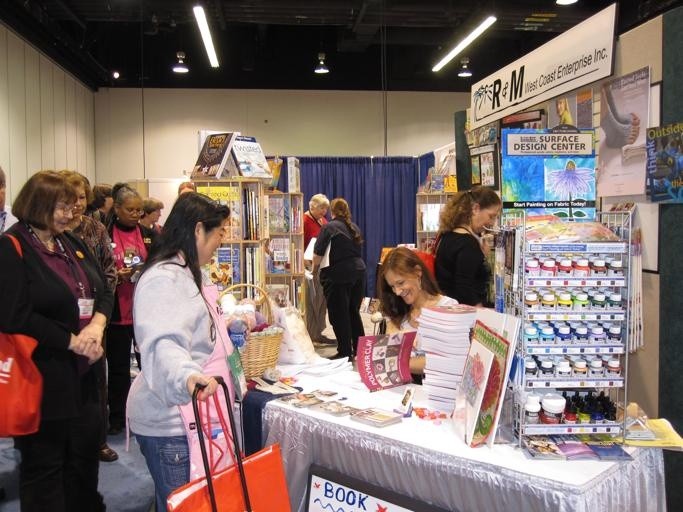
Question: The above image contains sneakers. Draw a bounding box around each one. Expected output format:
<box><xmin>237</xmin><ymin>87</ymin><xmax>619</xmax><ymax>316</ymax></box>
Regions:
<box><xmin>312</xmin><ymin>337</ymin><xmax>327</xmax><ymax>348</ymax></box>
<box><xmin>319</xmin><ymin>333</ymin><xmax>337</xmax><ymax>347</ymax></box>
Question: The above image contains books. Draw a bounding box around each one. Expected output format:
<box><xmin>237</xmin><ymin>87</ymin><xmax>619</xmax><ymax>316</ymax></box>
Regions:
<box><xmin>242</xmin><ymin>188</ymin><xmax>270</xmax><ymax>239</ymax></box>
<box><xmin>197</xmin><ymin>129</ymin><xmax>241</xmax><ymax>177</ymax></box>
<box><xmin>313</xmin><ymin>392</ymin><xmax>370</xmax><ymax>417</ymax></box>
<box><xmin>451</xmin><ymin>310</ymin><xmax>520</xmax><ymax>449</ymax></box>
<box><xmin>329</xmin><ymin>332</ymin><xmax>416</xmax><ymax>393</ymax></box>
<box><xmin>287</xmin><ymin>156</ymin><xmax>300</xmax><ymax>192</ymax></box>
<box><xmin>414</xmin><ymin>303</ymin><xmax>479</xmax><ymax>413</ymax></box>
<box><xmin>196</xmin><ymin>186</ymin><xmax>241</xmax><ymax>245</ymax></box>
<box><xmin>245</xmin><ymin>247</ymin><xmax>262</xmax><ymax>298</ymax></box>
<box><xmin>348</xmin><ymin>402</ymin><xmax>403</xmax><ymax>427</ymax></box>
<box><xmin>266</xmin><ymin>279</ymin><xmax>306</xmax><ymax>314</ymax></box>
<box><xmin>431</xmin><ymin>173</ymin><xmax>457</xmax><ymax>193</ymax></box>
<box><xmin>270</xmin><ymin>197</ymin><xmax>302</xmax><ymax>233</ymax></box>
<box><xmin>281</xmin><ymin>386</ymin><xmax>338</xmax><ymax>410</ymax></box>
<box><xmin>231</xmin><ymin>136</ymin><xmax>274</xmax><ymax>179</ymax></box>
<box><xmin>642</xmin><ymin>120</ymin><xmax>683</xmax><ymax>204</ymax></box>
<box><xmin>190</xmin><ymin>132</ymin><xmax>238</xmax><ymax>178</ymax></box>
<box><xmin>205</xmin><ymin>247</ymin><xmax>241</xmax><ymax>286</ymax></box>
<box><xmin>264</xmin><ymin>237</ymin><xmax>303</xmax><ymax>276</ymax></box>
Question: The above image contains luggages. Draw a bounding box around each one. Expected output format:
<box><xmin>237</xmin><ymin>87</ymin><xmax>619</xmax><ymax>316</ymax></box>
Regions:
<box><xmin>241</xmin><ymin>386</ymin><xmax>304</xmax><ymax>458</ymax></box>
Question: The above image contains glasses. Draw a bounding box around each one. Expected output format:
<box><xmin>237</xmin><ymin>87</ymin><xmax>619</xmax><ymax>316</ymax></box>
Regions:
<box><xmin>126</xmin><ymin>208</ymin><xmax>143</xmax><ymax>216</ymax></box>
<box><xmin>208</xmin><ymin>197</ymin><xmax>224</xmax><ymax>208</ymax></box>
<box><xmin>55</xmin><ymin>205</ymin><xmax>77</xmax><ymax>215</ymax></box>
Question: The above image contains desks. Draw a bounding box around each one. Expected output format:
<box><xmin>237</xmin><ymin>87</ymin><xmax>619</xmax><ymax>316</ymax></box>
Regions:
<box><xmin>232</xmin><ymin>359</ymin><xmax>667</xmax><ymax>510</ymax></box>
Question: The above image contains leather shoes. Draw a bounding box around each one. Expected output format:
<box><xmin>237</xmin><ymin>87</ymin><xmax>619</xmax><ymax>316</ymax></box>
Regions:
<box><xmin>98</xmin><ymin>443</ymin><xmax>118</xmax><ymax>462</ymax></box>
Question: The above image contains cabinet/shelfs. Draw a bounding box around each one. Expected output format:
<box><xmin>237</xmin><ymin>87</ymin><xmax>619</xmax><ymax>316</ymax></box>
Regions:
<box><xmin>516</xmin><ymin>207</ymin><xmax>633</xmax><ymax>449</ymax></box>
<box><xmin>415</xmin><ymin>190</ymin><xmax>458</xmax><ymax>252</ymax></box>
<box><xmin>178</xmin><ymin>179</ymin><xmax>263</xmax><ymax>304</ymax></box>
<box><xmin>264</xmin><ymin>190</ymin><xmax>306</xmax><ymax>329</ymax></box>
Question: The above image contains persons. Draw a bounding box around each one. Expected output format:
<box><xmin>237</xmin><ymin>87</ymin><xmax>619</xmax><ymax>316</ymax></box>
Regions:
<box><xmin>313</xmin><ymin>198</ymin><xmax>368</xmax><ymax>359</ymax></box>
<box><xmin>0</xmin><ymin>167</ymin><xmax>18</xmax><ymax>236</ymax></box>
<box><xmin>64</xmin><ymin>172</ymin><xmax>118</xmax><ymax>462</ymax></box>
<box><xmin>177</xmin><ymin>183</ymin><xmax>194</xmax><ymax>199</ymax></box>
<box><xmin>556</xmin><ymin>98</ymin><xmax>573</xmax><ymax>128</ymax></box>
<box><xmin>90</xmin><ymin>182</ymin><xmax>114</xmax><ymax>238</ymax></box>
<box><xmin>103</xmin><ymin>182</ymin><xmax>159</xmax><ymax>435</ymax></box>
<box><xmin>433</xmin><ymin>187</ymin><xmax>503</xmax><ymax>308</ymax></box>
<box><xmin>138</xmin><ymin>198</ymin><xmax>166</xmax><ymax>254</ymax></box>
<box><xmin>127</xmin><ymin>192</ymin><xmax>238</xmax><ymax>512</ymax></box>
<box><xmin>600</xmin><ymin>82</ymin><xmax>639</xmax><ymax>148</ymax></box>
<box><xmin>0</xmin><ymin>170</ymin><xmax>114</xmax><ymax>512</ymax></box>
<box><xmin>376</xmin><ymin>247</ymin><xmax>459</xmax><ymax>386</ymax></box>
<box><xmin>303</xmin><ymin>193</ymin><xmax>337</xmax><ymax>347</ymax></box>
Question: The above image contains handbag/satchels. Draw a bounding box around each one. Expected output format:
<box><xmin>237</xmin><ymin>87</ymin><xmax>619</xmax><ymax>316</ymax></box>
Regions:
<box><xmin>0</xmin><ymin>232</ymin><xmax>42</xmax><ymax>437</ymax></box>
<box><xmin>166</xmin><ymin>391</ymin><xmax>292</xmax><ymax>512</ymax></box>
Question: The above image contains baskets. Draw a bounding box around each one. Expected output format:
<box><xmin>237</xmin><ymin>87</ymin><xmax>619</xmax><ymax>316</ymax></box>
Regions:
<box><xmin>216</xmin><ymin>283</ymin><xmax>283</xmax><ymax>379</ymax></box>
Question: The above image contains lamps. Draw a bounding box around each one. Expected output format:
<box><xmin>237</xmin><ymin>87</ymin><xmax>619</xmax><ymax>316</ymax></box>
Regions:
<box><xmin>313</xmin><ymin>18</ymin><xmax>331</xmax><ymax>77</ymax></box>
<box><xmin>171</xmin><ymin>53</ymin><xmax>191</xmax><ymax>75</ymax></box>
<box><xmin>432</xmin><ymin>12</ymin><xmax>497</xmax><ymax>72</ymax></box>
<box><xmin>457</xmin><ymin>57</ymin><xmax>475</xmax><ymax>79</ymax></box>
<box><xmin>192</xmin><ymin>4</ymin><xmax>226</xmax><ymax>70</ymax></box>
